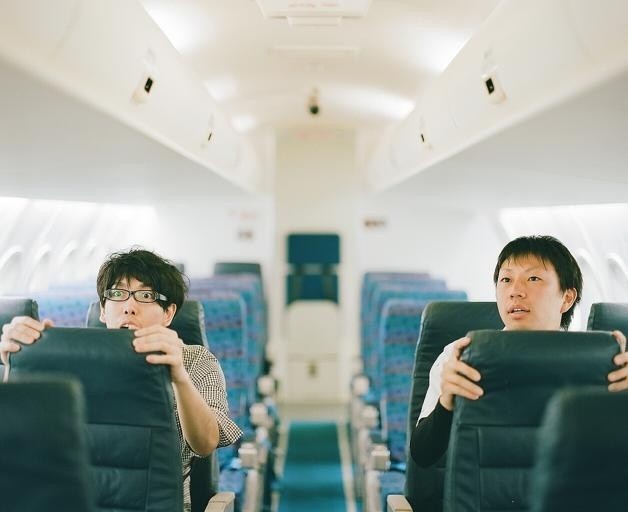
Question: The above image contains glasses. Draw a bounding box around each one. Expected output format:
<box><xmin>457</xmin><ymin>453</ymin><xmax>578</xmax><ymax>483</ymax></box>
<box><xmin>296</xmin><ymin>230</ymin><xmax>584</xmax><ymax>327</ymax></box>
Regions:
<box><xmin>103</xmin><ymin>288</ymin><xmax>168</xmax><ymax>303</ymax></box>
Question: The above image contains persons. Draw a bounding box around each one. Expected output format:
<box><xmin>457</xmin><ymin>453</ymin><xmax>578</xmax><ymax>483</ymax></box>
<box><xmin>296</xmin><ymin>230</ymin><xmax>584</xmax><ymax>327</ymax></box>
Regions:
<box><xmin>0</xmin><ymin>249</ymin><xmax>244</xmax><ymax>511</ymax></box>
<box><xmin>410</xmin><ymin>233</ymin><xmax>627</xmax><ymax>468</ymax></box>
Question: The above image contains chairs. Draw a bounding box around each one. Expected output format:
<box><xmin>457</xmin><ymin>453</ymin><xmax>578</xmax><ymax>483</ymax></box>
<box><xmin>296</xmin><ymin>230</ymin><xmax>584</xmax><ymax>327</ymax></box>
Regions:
<box><xmin>351</xmin><ymin>270</ymin><xmax>468</xmax><ymax>510</ymax></box>
<box><xmin>0</xmin><ymin>294</ymin><xmax>236</xmax><ymax>512</ymax></box>
<box><xmin>27</xmin><ymin>263</ymin><xmax>280</xmax><ymax>511</ymax></box>
<box><xmin>386</xmin><ymin>300</ymin><xmax>625</xmax><ymax>511</ymax></box>
